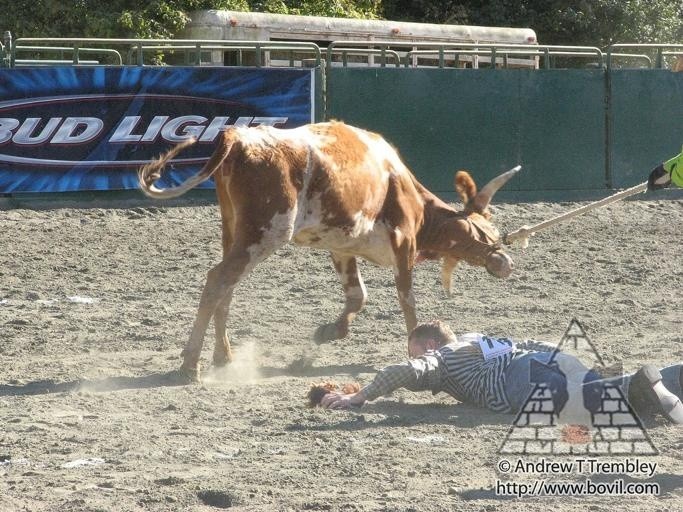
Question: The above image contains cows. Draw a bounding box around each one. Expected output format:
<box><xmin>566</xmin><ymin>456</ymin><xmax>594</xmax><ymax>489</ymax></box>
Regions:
<box><xmin>135</xmin><ymin>118</ymin><xmax>523</xmax><ymax>385</ymax></box>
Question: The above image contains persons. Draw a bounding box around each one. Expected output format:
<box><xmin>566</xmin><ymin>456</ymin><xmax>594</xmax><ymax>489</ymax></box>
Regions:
<box><xmin>648</xmin><ymin>150</ymin><xmax>683</xmax><ymax>191</ymax></box>
<box><xmin>319</xmin><ymin>320</ymin><xmax>683</xmax><ymax>428</ymax></box>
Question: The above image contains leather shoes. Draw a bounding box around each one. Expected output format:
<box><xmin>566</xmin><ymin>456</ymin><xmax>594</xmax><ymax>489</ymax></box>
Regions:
<box><xmin>633</xmin><ymin>363</ymin><xmax>682</xmax><ymax>425</ymax></box>
